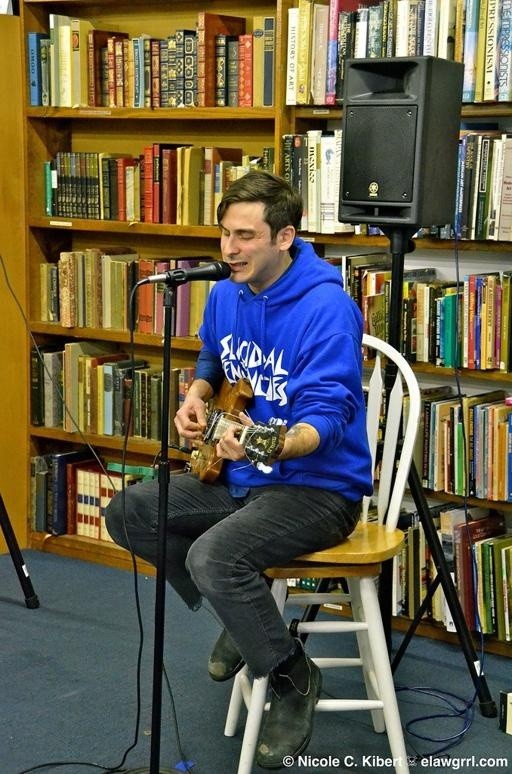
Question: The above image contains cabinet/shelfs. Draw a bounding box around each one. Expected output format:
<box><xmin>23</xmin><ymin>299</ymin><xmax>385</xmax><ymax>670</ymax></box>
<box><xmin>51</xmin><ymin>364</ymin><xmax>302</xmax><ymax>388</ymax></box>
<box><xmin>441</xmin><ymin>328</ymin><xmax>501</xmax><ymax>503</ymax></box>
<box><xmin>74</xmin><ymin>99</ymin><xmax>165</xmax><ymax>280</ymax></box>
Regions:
<box><xmin>275</xmin><ymin>2</ymin><xmax>512</xmax><ymax>658</ymax></box>
<box><xmin>27</xmin><ymin>2</ymin><xmax>281</xmax><ymax>577</ymax></box>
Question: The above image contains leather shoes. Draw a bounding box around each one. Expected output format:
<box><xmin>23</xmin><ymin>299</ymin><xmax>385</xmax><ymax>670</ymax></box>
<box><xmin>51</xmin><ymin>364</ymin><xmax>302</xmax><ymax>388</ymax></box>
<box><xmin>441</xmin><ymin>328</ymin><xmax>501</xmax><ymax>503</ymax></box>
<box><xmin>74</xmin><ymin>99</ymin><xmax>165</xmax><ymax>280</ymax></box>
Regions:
<box><xmin>254</xmin><ymin>636</ymin><xmax>323</xmax><ymax>770</ymax></box>
<box><xmin>207</xmin><ymin>576</ymin><xmax>289</xmax><ymax>682</ymax></box>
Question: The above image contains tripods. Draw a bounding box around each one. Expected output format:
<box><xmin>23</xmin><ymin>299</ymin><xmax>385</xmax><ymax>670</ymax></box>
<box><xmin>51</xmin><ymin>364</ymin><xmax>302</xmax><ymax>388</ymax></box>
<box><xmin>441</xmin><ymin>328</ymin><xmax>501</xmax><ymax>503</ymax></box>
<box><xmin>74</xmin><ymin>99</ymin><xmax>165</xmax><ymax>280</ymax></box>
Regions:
<box><xmin>297</xmin><ymin>224</ymin><xmax>499</xmax><ymax>719</ymax></box>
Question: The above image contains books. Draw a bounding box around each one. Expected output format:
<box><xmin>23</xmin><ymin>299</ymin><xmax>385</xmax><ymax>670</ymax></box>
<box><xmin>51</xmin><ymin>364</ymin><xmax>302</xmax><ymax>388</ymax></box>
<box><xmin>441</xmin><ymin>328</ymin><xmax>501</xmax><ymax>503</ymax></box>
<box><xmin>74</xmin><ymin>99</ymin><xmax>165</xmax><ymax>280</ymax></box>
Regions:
<box><xmin>393</xmin><ymin>508</ymin><xmax>511</xmax><ymax>646</ymax></box>
<box><xmin>286</xmin><ymin>576</ymin><xmax>320</xmax><ymax>590</ymax></box>
<box><xmin>322</xmin><ymin>251</ymin><xmax>512</xmax><ymax>373</ymax></box>
<box><xmin>364</xmin><ymin>385</ymin><xmax>512</xmax><ymax>504</ymax></box>
<box><xmin>43</xmin><ymin>141</ymin><xmax>276</xmax><ymax>227</ymax></box>
<box><xmin>25</xmin><ymin>3</ymin><xmax>282</xmax><ymax>109</ymax></box>
<box><xmin>27</xmin><ymin>450</ymin><xmax>142</xmax><ymax>543</ymax></box>
<box><xmin>414</xmin><ymin>128</ymin><xmax>512</xmax><ymax>242</ymax></box>
<box><xmin>28</xmin><ymin>340</ymin><xmax>197</xmax><ymax>451</ymax></box>
<box><xmin>38</xmin><ymin>247</ymin><xmax>220</xmax><ymax>338</ymax></box>
<box><xmin>283</xmin><ymin>0</ymin><xmax>511</xmax><ymax>104</ymax></box>
<box><xmin>277</xmin><ymin>128</ymin><xmax>353</xmax><ymax>234</ymax></box>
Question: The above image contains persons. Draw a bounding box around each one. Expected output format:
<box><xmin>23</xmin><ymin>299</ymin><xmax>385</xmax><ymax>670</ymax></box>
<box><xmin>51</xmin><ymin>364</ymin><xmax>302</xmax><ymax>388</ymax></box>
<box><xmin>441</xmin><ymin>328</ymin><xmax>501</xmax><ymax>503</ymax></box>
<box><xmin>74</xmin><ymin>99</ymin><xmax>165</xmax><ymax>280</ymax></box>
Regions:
<box><xmin>103</xmin><ymin>170</ymin><xmax>376</xmax><ymax>770</ymax></box>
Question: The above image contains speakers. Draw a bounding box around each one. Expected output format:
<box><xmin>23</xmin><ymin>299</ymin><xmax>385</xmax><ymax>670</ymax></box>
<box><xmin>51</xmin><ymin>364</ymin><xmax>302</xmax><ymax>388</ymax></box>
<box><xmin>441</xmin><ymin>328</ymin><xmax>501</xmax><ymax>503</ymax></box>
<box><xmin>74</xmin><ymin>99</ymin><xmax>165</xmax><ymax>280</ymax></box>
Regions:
<box><xmin>339</xmin><ymin>56</ymin><xmax>465</xmax><ymax>227</ymax></box>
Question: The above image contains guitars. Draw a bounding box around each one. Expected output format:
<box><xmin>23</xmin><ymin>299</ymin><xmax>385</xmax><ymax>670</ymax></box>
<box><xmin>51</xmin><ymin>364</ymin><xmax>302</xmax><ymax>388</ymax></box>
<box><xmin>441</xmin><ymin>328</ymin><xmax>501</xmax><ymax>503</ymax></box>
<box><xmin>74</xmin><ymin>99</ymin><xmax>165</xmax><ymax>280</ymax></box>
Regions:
<box><xmin>182</xmin><ymin>376</ymin><xmax>288</xmax><ymax>485</ymax></box>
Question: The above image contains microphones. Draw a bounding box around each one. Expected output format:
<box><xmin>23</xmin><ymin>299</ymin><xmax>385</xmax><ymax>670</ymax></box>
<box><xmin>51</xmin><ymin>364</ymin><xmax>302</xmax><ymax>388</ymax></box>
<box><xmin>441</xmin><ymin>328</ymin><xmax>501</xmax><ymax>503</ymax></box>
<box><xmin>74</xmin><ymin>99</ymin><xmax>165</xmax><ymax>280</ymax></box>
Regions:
<box><xmin>139</xmin><ymin>261</ymin><xmax>232</xmax><ymax>285</ymax></box>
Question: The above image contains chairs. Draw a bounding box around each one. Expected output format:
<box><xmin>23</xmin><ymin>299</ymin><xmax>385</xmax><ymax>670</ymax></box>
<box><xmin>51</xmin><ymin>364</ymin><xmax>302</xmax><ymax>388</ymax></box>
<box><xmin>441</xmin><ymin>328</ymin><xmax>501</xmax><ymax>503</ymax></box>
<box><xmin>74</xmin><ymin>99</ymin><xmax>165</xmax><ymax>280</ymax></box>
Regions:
<box><xmin>224</xmin><ymin>334</ymin><xmax>422</xmax><ymax>772</ymax></box>
